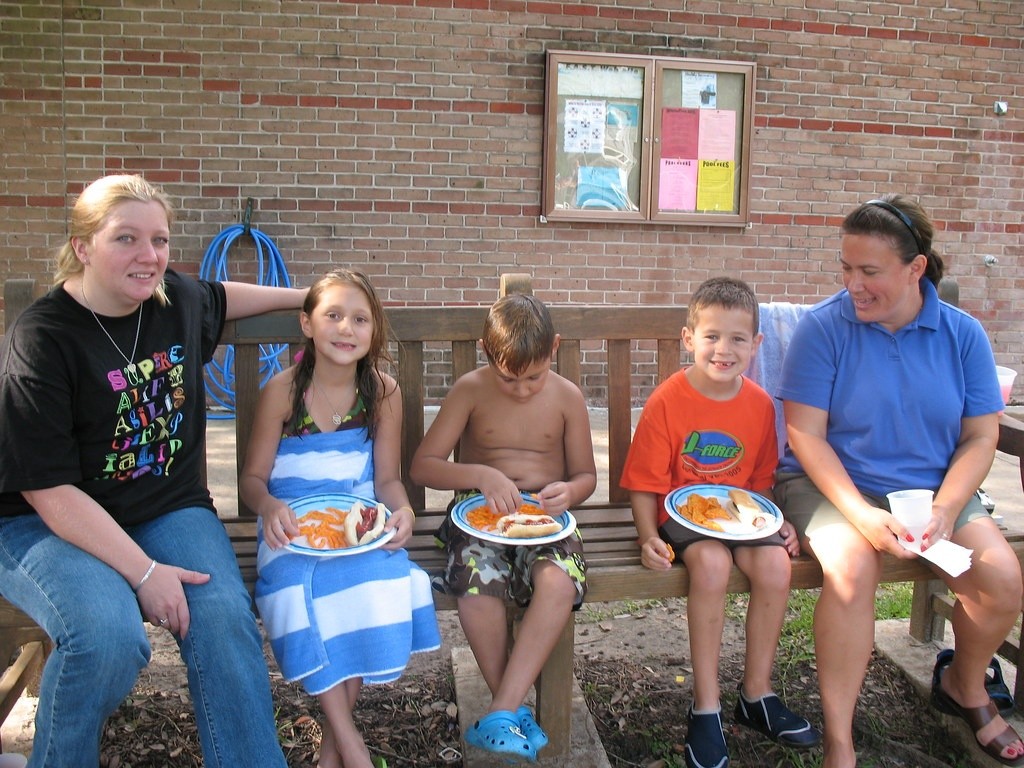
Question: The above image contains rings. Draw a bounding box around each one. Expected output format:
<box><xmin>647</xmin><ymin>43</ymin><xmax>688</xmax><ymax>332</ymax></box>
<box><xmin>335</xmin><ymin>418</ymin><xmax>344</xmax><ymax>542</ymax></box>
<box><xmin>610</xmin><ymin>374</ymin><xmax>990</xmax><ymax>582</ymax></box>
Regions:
<box><xmin>159</xmin><ymin>618</ymin><xmax>168</xmax><ymax>627</ymax></box>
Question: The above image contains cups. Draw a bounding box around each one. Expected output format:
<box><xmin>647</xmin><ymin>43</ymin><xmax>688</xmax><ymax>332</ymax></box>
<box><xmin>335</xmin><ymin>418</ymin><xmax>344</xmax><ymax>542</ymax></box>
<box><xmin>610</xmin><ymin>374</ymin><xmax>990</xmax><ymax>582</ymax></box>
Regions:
<box><xmin>886</xmin><ymin>489</ymin><xmax>935</xmax><ymax>546</ymax></box>
<box><xmin>996</xmin><ymin>365</ymin><xmax>1017</xmax><ymax>416</ymax></box>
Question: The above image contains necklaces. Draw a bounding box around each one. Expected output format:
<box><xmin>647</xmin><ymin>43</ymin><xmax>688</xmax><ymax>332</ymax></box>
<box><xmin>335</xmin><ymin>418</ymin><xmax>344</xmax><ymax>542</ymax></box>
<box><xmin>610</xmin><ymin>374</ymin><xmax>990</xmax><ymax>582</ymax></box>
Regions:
<box><xmin>314</xmin><ymin>373</ymin><xmax>366</xmax><ymax>447</ymax></box>
<box><xmin>76</xmin><ymin>278</ymin><xmax>145</xmax><ymax>391</ymax></box>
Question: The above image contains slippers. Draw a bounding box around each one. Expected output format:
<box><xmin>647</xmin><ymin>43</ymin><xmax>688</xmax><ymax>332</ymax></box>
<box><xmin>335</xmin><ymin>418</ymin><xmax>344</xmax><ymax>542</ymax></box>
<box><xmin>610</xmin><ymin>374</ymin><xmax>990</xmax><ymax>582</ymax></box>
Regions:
<box><xmin>935</xmin><ymin>683</ymin><xmax>1024</xmax><ymax>766</ymax></box>
<box><xmin>464</xmin><ymin>710</ymin><xmax>536</xmax><ymax>760</ymax></box>
<box><xmin>512</xmin><ymin>706</ymin><xmax>549</xmax><ymax>749</ymax></box>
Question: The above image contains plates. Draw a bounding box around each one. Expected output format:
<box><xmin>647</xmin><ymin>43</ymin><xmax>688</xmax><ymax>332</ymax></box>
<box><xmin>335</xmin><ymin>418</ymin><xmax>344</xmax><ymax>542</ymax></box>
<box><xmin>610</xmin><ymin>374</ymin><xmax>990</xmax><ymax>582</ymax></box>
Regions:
<box><xmin>280</xmin><ymin>493</ymin><xmax>397</xmax><ymax>557</ymax></box>
<box><xmin>664</xmin><ymin>484</ymin><xmax>784</xmax><ymax>540</ymax></box>
<box><xmin>451</xmin><ymin>493</ymin><xmax>576</xmax><ymax>545</ymax></box>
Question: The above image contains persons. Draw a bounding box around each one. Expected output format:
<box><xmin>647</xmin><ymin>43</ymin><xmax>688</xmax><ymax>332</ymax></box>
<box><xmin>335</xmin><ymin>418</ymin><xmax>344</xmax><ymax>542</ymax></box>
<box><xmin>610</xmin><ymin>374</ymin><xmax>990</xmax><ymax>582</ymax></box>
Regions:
<box><xmin>620</xmin><ymin>276</ymin><xmax>819</xmax><ymax>768</ymax></box>
<box><xmin>412</xmin><ymin>291</ymin><xmax>596</xmax><ymax>761</ymax></box>
<box><xmin>236</xmin><ymin>268</ymin><xmax>420</xmax><ymax>768</ymax></box>
<box><xmin>772</xmin><ymin>194</ymin><xmax>1018</xmax><ymax>768</ymax></box>
<box><xmin>2</xmin><ymin>174</ymin><xmax>314</xmax><ymax>767</ymax></box>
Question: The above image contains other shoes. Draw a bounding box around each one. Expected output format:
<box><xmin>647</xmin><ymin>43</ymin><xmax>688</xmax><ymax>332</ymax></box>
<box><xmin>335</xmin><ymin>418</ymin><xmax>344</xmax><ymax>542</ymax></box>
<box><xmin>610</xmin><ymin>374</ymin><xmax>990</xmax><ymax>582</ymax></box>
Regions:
<box><xmin>684</xmin><ymin>703</ymin><xmax>730</xmax><ymax>768</ymax></box>
<box><xmin>732</xmin><ymin>682</ymin><xmax>820</xmax><ymax>746</ymax></box>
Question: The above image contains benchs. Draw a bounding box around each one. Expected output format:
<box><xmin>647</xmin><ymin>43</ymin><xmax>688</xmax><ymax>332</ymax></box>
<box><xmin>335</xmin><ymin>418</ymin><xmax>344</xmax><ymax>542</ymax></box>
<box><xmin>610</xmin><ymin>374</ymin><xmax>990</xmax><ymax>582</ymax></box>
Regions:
<box><xmin>0</xmin><ymin>273</ymin><xmax>1024</xmax><ymax>768</ymax></box>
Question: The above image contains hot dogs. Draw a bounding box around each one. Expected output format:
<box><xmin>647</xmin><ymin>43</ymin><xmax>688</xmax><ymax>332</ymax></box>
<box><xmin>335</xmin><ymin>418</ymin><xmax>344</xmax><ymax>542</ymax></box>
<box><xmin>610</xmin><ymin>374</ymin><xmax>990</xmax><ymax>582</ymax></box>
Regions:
<box><xmin>727</xmin><ymin>490</ymin><xmax>767</xmax><ymax>529</ymax></box>
<box><xmin>344</xmin><ymin>501</ymin><xmax>385</xmax><ymax>546</ymax></box>
<box><xmin>496</xmin><ymin>516</ymin><xmax>562</xmax><ymax>538</ymax></box>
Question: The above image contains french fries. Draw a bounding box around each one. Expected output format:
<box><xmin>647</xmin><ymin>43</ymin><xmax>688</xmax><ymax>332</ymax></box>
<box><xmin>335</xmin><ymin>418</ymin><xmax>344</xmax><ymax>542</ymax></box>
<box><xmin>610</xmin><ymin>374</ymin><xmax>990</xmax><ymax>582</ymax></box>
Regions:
<box><xmin>466</xmin><ymin>504</ymin><xmax>547</xmax><ymax>531</ymax></box>
<box><xmin>284</xmin><ymin>508</ymin><xmax>347</xmax><ymax>550</ymax></box>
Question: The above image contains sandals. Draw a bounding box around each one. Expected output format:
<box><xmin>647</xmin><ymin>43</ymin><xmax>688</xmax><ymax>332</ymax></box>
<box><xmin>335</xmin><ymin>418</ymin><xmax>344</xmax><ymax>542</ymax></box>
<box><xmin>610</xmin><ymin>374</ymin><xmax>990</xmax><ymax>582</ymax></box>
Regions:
<box><xmin>983</xmin><ymin>655</ymin><xmax>1016</xmax><ymax>718</ymax></box>
<box><xmin>931</xmin><ymin>647</ymin><xmax>960</xmax><ymax>715</ymax></box>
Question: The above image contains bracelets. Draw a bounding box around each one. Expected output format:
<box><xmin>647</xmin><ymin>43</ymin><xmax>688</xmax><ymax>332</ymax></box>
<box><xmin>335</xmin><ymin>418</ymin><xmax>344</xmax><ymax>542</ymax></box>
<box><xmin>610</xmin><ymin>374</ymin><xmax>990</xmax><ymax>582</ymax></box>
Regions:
<box><xmin>399</xmin><ymin>504</ymin><xmax>418</xmax><ymax>526</ymax></box>
<box><xmin>132</xmin><ymin>559</ymin><xmax>159</xmax><ymax>594</ymax></box>
